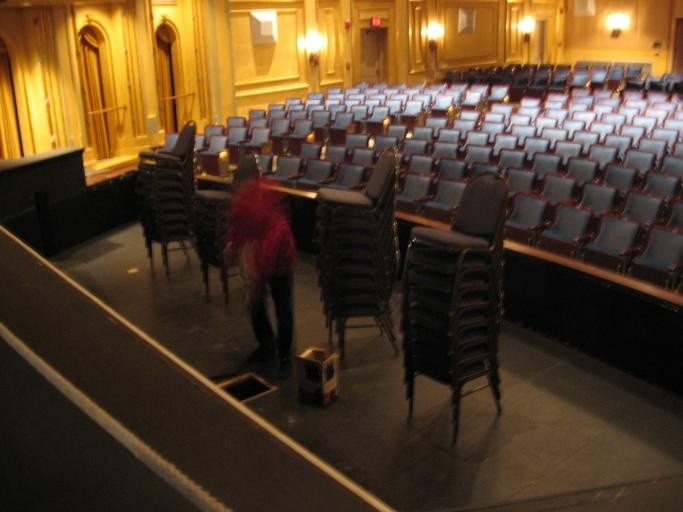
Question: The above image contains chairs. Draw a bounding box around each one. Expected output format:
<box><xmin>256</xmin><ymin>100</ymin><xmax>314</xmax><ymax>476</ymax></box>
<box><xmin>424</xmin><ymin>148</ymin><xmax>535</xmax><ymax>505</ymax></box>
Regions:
<box><xmin>135</xmin><ymin>61</ymin><xmax>683</xmax><ymax>441</ymax></box>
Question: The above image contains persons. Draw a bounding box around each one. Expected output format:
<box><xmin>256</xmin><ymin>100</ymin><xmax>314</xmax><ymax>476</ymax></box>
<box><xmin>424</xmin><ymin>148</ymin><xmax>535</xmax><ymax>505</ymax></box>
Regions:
<box><xmin>220</xmin><ymin>151</ymin><xmax>299</xmax><ymax>379</ymax></box>
